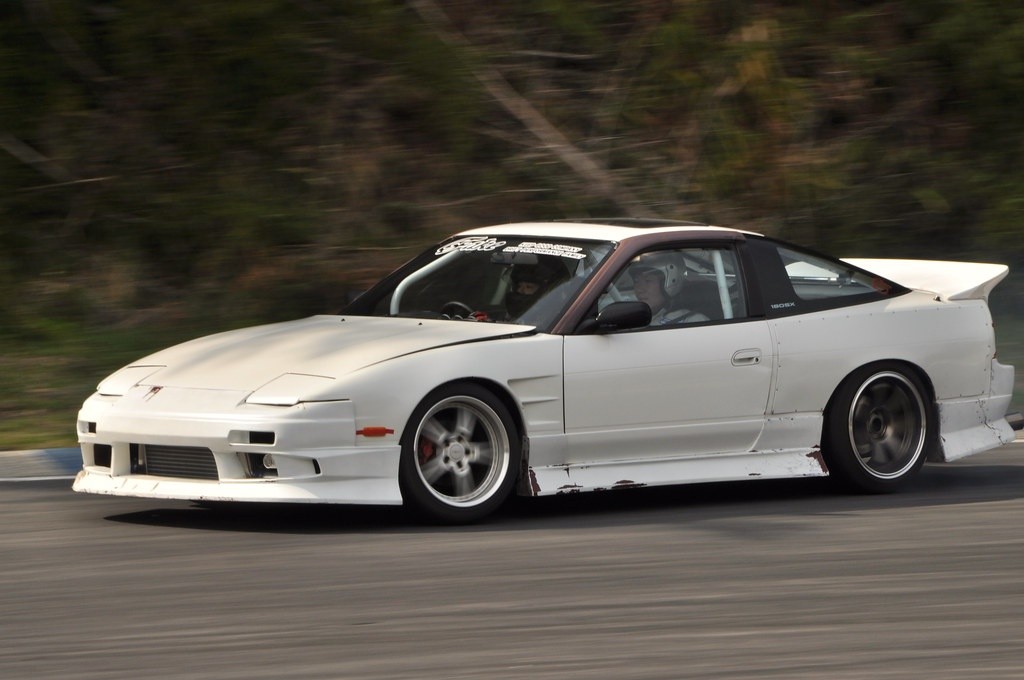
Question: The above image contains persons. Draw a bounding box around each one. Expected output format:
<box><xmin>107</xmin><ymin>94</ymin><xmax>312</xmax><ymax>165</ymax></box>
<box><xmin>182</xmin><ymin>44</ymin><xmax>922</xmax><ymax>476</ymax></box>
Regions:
<box><xmin>596</xmin><ymin>246</ymin><xmax>712</xmax><ymax>334</ymax></box>
<box><xmin>470</xmin><ymin>255</ymin><xmax>573</xmax><ymax>325</ymax></box>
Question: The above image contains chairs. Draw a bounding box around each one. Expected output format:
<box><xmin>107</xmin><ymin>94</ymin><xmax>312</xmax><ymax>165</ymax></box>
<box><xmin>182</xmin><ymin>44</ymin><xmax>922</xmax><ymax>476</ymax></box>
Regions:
<box><xmin>672</xmin><ymin>281</ymin><xmax>724</xmax><ymax>319</ymax></box>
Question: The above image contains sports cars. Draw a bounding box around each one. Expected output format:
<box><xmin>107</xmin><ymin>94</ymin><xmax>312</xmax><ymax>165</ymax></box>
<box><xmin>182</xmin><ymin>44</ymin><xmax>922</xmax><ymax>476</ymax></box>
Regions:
<box><xmin>69</xmin><ymin>215</ymin><xmax>1017</xmax><ymax>528</ymax></box>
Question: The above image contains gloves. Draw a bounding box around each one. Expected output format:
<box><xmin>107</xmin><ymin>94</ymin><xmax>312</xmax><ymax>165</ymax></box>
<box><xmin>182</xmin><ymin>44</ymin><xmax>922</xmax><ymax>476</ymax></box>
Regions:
<box><xmin>468</xmin><ymin>311</ymin><xmax>496</xmax><ymax>323</ymax></box>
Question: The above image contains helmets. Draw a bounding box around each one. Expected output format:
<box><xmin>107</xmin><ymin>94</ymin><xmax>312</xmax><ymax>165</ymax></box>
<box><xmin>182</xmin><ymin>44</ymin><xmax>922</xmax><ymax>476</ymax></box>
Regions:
<box><xmin>501</xmin><ymin>254</ymin><xmax>572</xmax><ymax>317</ymax></box>
<box><xmin>628</xmin><ymin>249</ymin><xmax>687</xmax><ymax>299</ymax></box>
